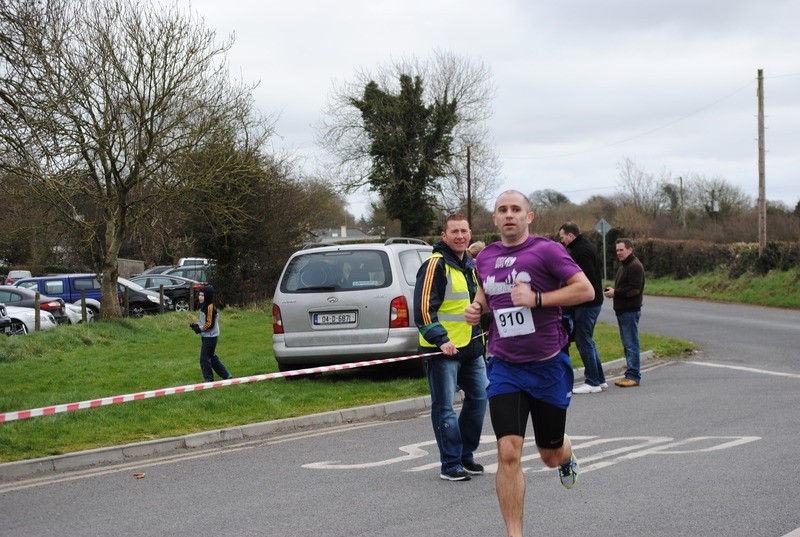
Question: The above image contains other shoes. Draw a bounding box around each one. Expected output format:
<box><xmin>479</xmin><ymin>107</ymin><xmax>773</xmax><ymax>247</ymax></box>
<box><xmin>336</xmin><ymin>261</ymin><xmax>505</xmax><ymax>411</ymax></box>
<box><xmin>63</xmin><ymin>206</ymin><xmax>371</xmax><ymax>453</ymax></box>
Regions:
<box><xmin>615</xmin><ymin>377</ymin><xmax>639</xmax><ymax>386</ymax></box>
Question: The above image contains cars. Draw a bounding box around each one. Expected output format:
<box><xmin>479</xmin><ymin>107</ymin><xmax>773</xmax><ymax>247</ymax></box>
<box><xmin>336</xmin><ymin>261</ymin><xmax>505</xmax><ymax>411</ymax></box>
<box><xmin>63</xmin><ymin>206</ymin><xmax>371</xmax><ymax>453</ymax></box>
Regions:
<box><xmin>0</xmin><ymin>285</ymin><xmax>69</xmax><ymax>324</ymax></box>
<box><xmin>125</xmin><ymin>257</ymin><xmax>219</xmax><ymax>313</ymax></box>
<box><xmin>0</xmin><ymin>302</ymin><xmax>58</xmax><ymax>336</ymax></box>
<box><xmin>292</xmin><ymin>226</ymin><xmax>375</xmax><ymax>248</ymax></box>
<box><xmin>65</xmin><ymin>304</ymin><xmax>94</xmax><ymax>326</ymax></box>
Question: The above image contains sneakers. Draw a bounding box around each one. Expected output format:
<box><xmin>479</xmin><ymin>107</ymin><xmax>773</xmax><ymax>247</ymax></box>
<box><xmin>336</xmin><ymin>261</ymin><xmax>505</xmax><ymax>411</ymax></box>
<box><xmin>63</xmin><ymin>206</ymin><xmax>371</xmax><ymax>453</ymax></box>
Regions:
<box><xmin>441</xmin><ymin>466</ymin><xmax>472</xmax><ymax>481</ymax></box>
<box><xmin>573</xmin><ymin>384</ymin><xmax>602</xmax><ymax>394</ymax></box>
<box><xmin>461</xmin><ymin>460</ymin><xmax>483</xmax><ymax>475</ymax></box>
<box><xmin>558</xmin><ymin>434</ymin><xmax>579</xmax><ymax>489</ymax></box>
<box><xmin>600</xmin><ymin>383</ymin><xmax>608</xmax><ymax>390</ymax></box>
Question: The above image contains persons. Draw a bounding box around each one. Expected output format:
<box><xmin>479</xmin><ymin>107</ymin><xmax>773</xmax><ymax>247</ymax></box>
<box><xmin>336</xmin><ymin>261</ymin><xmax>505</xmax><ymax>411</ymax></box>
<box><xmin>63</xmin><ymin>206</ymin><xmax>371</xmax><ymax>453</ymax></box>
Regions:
<box><xmin>558</xmin><ymin>222</ymin><xmax>645</xmax><ymax>394</ymax></box>
<box><xmin>467</xmin><ymin>241</ymin><xmax>486</xmax><ymax>265</ymax></box>
<box><xmin>463</xmin><ymin>189</ymin><xmax>596</xmax><ymax>537</ymax></box>
<box><xmin>413</xmin><ymin>215</ymin><xmax>491</xmax><ymax>481</ymax></box>
<box><xmin>189</xmin><ymin>286</ymin><xmax>233</xmax><ymax>383</ymax></box>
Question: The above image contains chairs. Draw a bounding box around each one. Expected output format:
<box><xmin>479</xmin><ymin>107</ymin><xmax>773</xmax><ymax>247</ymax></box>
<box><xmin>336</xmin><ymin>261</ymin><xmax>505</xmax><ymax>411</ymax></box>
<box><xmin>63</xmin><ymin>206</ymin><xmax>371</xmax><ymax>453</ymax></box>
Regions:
<box><xmin>349</xmin><ymin>270</ymin><xmax>370</xmax><ymax>287</ymax></box>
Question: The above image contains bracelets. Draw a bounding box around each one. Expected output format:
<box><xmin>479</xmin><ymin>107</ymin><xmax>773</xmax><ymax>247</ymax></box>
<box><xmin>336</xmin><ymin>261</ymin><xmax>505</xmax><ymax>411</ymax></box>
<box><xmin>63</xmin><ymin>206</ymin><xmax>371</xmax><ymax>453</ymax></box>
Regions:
<box><xmin>536</xmin><ymin>291</ymin><xmax>541</xmax><ymax>310</ymax></box>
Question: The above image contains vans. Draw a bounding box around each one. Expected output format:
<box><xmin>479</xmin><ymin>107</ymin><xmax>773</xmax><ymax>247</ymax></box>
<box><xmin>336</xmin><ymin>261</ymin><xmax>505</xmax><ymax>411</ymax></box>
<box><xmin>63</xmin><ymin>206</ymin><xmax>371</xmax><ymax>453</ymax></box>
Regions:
<box><xmin>11</xmin><ymin>272</ymin><xmax>174</xmax><ymax>318</ymax></box>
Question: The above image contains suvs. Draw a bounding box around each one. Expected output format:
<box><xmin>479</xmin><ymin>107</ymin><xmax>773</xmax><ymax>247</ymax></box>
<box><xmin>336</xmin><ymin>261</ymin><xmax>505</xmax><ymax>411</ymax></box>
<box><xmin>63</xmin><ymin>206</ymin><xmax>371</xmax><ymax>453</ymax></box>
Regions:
<box><xmin>270</xmin><ymin>237</ymin><xmax>431</xmax><ymax>383</ymax></box>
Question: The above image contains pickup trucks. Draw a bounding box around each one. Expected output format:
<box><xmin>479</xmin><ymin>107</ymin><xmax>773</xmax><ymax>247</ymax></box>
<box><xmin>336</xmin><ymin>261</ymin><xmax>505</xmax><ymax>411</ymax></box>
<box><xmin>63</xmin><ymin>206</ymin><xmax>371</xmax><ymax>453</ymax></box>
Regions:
<box><xmin>5</xmin><ymin>270</ymin><xmax>32</xmax><ymax>286</ymax></box>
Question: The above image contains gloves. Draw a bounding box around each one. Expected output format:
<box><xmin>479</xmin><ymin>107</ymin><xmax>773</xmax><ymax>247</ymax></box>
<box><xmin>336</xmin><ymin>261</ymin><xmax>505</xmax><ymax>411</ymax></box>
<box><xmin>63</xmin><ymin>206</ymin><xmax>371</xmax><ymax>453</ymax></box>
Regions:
<box><xmin>193</xmin><ymin>327</ymin><xmax>202</xmax><ymax>334</ymax></box>
<box><xmin>189</xmin><ymin>323</ymin><xmax>199</xmax><ymax>330</ymax></box>
